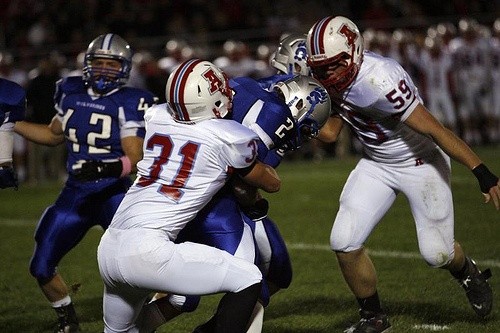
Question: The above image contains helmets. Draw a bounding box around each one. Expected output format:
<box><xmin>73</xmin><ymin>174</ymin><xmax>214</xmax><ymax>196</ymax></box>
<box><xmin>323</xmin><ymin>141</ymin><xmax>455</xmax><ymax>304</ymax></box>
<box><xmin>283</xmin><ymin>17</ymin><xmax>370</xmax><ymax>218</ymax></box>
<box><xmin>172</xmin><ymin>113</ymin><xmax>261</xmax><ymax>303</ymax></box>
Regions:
<box><xmin>271</xmin><ymin>34</ymin><xmax>312</xmax><ymax>77</ymax></box>
<box><xmin>83</xmin><ymin>33</ymin><xmax>132</xmax><ymax>94</ymax></box>
<box><xmin>166</xmin><ymin>57</ymin><xmax>235</xmax><ymax>125</ymax></box>
<box><xmin>307</xmin><ymin>16</ymin><xmax>363</xmax><ymax>91</ymax></box>
<box><xmin>273</xmin><ymin>76</ymin><xmax>331</xmax><ymax>138</ymax></box>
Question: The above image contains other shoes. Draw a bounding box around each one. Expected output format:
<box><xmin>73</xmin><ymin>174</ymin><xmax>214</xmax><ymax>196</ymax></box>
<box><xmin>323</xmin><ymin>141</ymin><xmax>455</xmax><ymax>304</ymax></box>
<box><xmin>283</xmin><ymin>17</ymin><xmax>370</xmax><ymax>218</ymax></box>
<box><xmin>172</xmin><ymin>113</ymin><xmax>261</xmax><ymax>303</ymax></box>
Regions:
<box><xmin>52</xmin><ymin>301</ymin><xmax>79</xmax><ymax>332</ymax></box>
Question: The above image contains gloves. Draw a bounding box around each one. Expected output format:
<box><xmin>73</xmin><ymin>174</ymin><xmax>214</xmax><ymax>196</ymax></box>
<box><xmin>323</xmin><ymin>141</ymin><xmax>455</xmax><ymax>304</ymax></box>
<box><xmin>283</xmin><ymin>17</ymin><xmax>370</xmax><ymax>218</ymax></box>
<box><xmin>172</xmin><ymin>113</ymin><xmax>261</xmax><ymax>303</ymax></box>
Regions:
<box><xmin>73</xmin><ymin>158</ymin><xmax>123</xmax><ymax>181</ymax></box>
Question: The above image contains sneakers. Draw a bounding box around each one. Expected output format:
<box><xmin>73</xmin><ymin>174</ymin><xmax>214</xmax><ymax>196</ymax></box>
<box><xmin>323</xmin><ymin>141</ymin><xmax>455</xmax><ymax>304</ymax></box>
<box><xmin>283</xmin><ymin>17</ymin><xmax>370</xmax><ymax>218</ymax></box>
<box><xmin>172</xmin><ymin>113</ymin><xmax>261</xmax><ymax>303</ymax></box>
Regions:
<box><xmin>343</xmin><ymin>308</ymin><xmax>393</xmax><ymax>333</ymax></box>
<box><xmin>460</xmin><ymin>258</ymin><xmax>493</xmax><ymax>316</ymax></box>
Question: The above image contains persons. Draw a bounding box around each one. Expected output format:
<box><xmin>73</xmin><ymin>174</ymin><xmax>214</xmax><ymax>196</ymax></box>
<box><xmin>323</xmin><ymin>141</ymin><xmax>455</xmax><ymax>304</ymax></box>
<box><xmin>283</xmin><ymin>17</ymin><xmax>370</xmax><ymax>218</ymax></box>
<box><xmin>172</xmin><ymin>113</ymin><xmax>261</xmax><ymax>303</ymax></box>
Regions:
<box><xmin>305</xmin><ymin>15</ymin><xmax>500</xmax><ymax>333</ymax></box>
<box><xmin>0</xmin><ymin>33</ymin><xmax>159</xmax><ymax>333</ymax></box>
<box><xmin>95</xmin><ymin>33</ymin><xmax>332</xmax><ymax>333</ymax></box>
<box><xmin>324</xmin><ymin>17</ymin><xmax>500</xmax><ymax>159</ymax></box>
<box><xmin>0</xmin><ymin>25</ymin><xmax>90</xmax><ymax>190</ymax></box>
